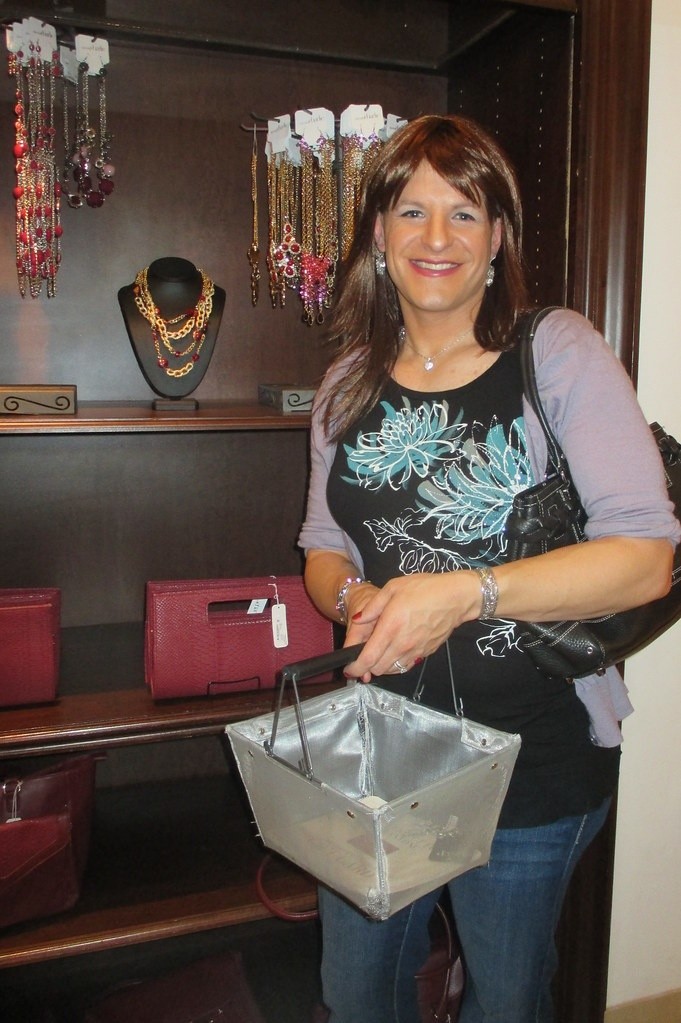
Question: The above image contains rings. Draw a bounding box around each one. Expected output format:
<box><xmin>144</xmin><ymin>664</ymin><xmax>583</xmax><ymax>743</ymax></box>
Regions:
<box><xmin>394</xmin><ymin>660</ymin><xmax>405</xmax><ymax>676</ymax></box>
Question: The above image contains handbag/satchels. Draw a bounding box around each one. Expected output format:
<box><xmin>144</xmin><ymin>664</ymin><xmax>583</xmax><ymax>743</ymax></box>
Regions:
<box><xmin>39</xmin><ymin>944</ymin><xmax>270</xmax><ymax>1023</ymax></box>
<box><xmin>0</xmin><ymin>587</ymin><xmax>62</xmax><ymax>709</ymax></box>
<box><xmin>1</xmin><ymin>751</ymin><xmax>109</xmax><ymax>928</ymax></box>
<box><xmin>308</xmin><ymin>901</ymin><xmax>465</xmax><ymax>1023</ymax></box>
<box><xmin>504</xmin><ymin>305</ymin><xmax>681</xmax><ymax>685</ymax></box>
<box><xmin>144</xmin><ymin>576</ymin><xmax>334</xmax><ymax>700</ymax></box>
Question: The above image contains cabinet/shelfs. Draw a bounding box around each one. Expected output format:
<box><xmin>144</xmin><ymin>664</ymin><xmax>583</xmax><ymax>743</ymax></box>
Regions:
<box><xmin>1</xmin><ymin>399</ymin><xmax>347</xmax><ymax>958</ymax></box>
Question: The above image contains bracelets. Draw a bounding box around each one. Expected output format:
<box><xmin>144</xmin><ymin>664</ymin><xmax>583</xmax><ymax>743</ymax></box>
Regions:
<box><xmin>335</xmin><ymin>577</ymin><xmax>370</xmax><ymax>628</ymax></box>
<box><xmin>474</xmin><ymin>564</ymin><xmax>499</xmax><ymax>623</ymax></box>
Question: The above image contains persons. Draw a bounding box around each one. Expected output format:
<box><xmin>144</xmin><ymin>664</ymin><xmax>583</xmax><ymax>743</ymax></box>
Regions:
<box><xmin>297</xmin><ymin>114</ymin><xmax>680</xmax><ymax>1023</ymax></box>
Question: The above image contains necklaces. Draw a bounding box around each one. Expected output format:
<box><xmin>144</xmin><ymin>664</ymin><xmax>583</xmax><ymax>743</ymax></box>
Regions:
<box><xmin>7</xmin><ymin>43</ymin><xmax>396</xmax><ymax>380</ymax></box>
<box><xmin>397</xmin><ymin>324</ymin><xmax>479</xmax><ymax>371</ymax></box>
